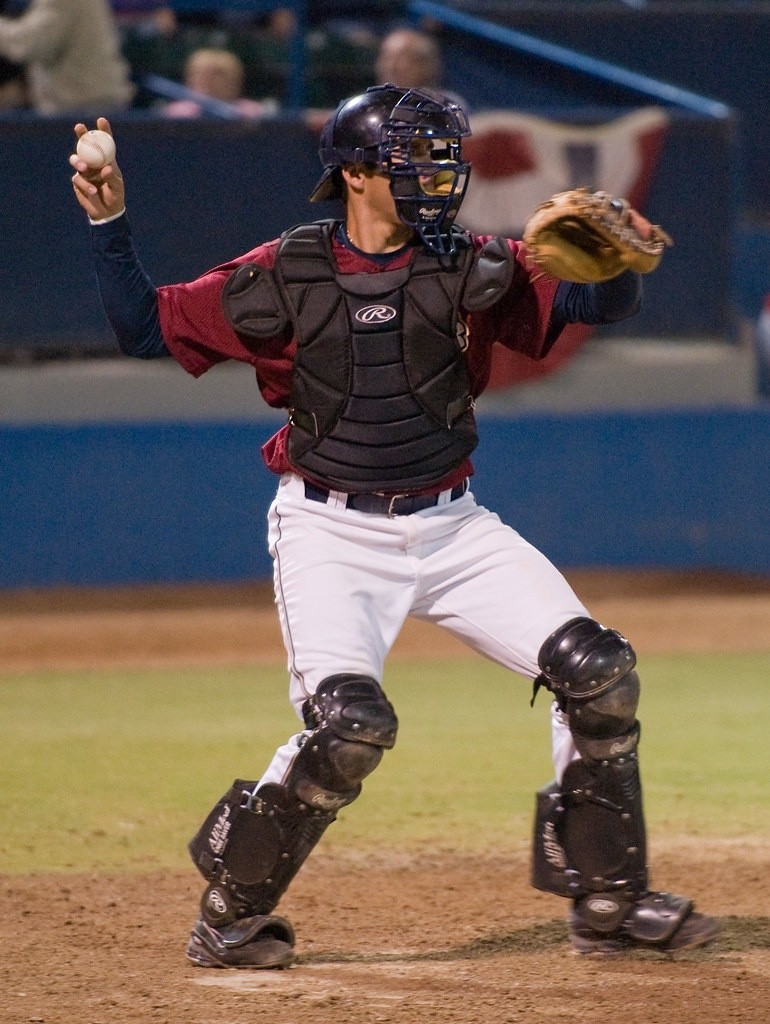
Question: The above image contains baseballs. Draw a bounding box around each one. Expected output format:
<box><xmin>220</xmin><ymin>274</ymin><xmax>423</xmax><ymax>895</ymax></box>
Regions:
<box><xmin>73</xmin><ymin>128</ymin><xmax>118</xmax><ymax>170</ymax></box>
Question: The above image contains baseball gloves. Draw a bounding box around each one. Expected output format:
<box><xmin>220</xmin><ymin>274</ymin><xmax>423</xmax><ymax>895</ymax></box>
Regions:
<box><xmin>526</xmin><ymin>181</ymin><xmax>675</xmax><ymax>288</ymax></box>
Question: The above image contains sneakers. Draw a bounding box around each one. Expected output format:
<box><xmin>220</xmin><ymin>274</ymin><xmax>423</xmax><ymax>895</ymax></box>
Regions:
<box><xmin>185</xmin><ymin>915</ymin><xmax>298</xmax><ymax>970</ymax></box>
<box><xmin>566</xmin><ymin>891</ymin><xmax>727</xmax><ymax>956</ymax></box>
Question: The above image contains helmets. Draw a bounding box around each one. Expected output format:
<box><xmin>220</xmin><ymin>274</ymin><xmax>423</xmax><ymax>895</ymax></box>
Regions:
<box><xmin>307</xmin><ymin>82</ymin><xmax>473</xmax><ymax>204</ymax></box>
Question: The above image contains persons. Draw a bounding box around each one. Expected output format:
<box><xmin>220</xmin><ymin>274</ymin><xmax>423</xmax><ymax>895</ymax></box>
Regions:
<box><xmin>69</xmin><ymin>84</ymin><xmax>724</xmax><ymax>973</ymax></box>
<box><xmin>0</xmin><ymin>0</ymin><xmax>469</xmax><ymax>118</ymax></box>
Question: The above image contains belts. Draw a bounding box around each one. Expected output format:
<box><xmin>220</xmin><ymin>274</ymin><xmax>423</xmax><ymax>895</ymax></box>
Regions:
<box><xmin>304</xmin><ymin>479</ymin><xmax>467</xmax><ymax>516</ymax></box>
